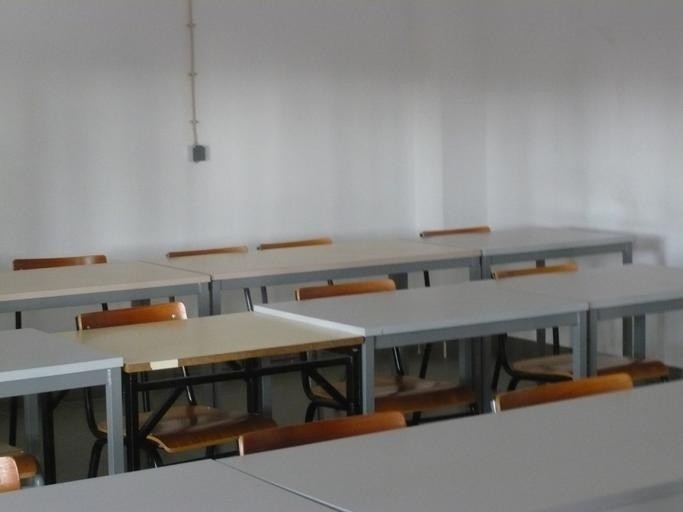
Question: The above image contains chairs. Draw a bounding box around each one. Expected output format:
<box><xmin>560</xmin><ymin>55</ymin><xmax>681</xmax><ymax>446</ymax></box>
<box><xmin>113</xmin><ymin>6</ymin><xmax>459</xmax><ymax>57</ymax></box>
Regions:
<box><xmin>0</xmin><ymin>226</ymin><xmax>683</xmax><ymax>492</ymax></box>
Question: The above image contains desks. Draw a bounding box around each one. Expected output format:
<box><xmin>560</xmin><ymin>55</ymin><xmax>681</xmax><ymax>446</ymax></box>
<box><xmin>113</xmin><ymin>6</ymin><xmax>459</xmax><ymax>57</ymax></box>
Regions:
<box><xmin>0</xmin><ymin>379</ymin><xmax>683</xmax><ymax>512</ymax></box>
